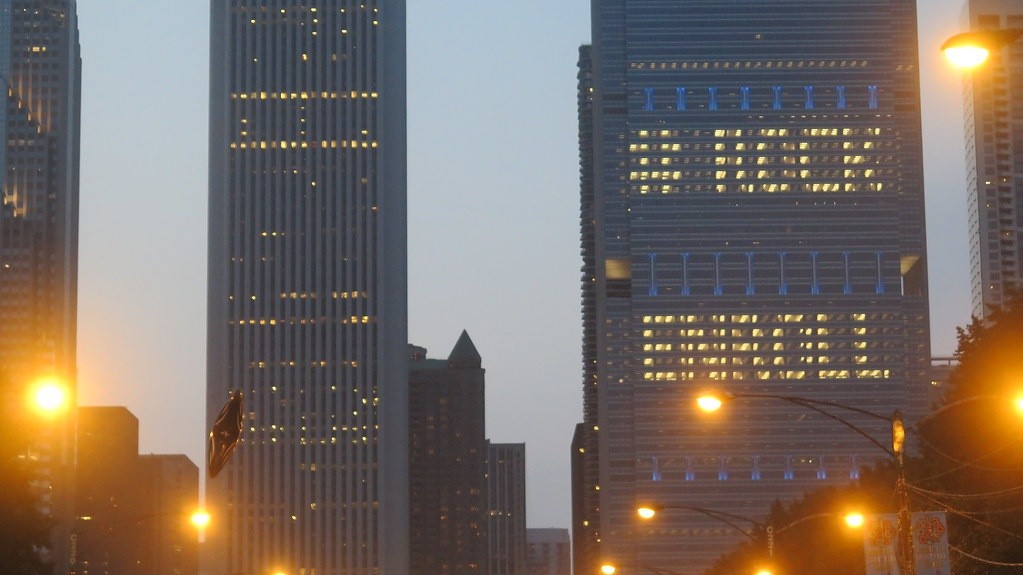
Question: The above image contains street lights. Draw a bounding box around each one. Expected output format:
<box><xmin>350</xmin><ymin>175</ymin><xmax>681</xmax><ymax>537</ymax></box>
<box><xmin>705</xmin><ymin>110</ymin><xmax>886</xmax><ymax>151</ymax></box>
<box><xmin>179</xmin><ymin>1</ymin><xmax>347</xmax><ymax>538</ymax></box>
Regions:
<box><xmin>32</xmin><ymin>370</ymin><xmax>211</xmax><ymax>575</ymax></box>
<box><xmin>695</xmin><ymin>389</ymin><xmax>1023</xmax><ymax>575</ymax></box>
<box><xmin>636</xmin><ymin>503</ymin><xmax>867</xmax><ymax>575</ymax></box>
